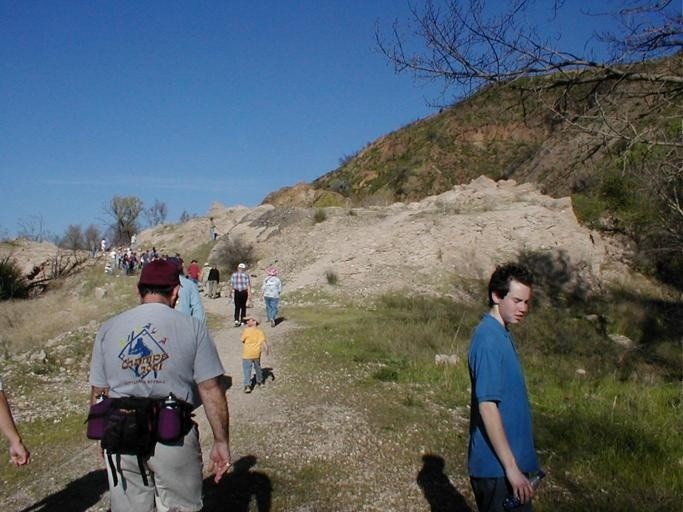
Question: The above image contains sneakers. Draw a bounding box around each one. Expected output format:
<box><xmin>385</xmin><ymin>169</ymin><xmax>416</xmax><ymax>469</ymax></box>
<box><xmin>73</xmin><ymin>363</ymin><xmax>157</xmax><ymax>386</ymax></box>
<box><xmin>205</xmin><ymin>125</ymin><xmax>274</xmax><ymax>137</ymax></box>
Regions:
<box><xmin>265</xmin><ymin>318</ymin><xmax>277</xmax><ymax>327</ymax></box>
<box><xmin>234</xmin><ymin>318</ymin><xmax>246</xmax><ymax>326</ymax></box>
<box><xmin>244</xmin><ymin>385</ymin><xmax>253</xmax><ymax>393</ymax></box>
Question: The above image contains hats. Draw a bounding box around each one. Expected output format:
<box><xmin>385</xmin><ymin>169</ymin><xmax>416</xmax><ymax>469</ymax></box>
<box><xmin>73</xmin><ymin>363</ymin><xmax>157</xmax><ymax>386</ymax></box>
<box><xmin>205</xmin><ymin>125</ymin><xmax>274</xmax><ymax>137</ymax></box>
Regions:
<box><xmin>243</xmin><ymin>313</ymin><xmax>261</xmax><ymax>324</ymax></box>
<box><xmin>238</xmin><ymin>263</ymin><xmax>246</xmax><ymax>269</ymax></box>
<box><xmin>269</xmin><ymin>269</ymin><xmax>278</xmax><ymax>275</ymax></box>
<box><xmin>140</xmin><ymin>260</ymin><xmax>184</xmax><ymax>290</ymax></box>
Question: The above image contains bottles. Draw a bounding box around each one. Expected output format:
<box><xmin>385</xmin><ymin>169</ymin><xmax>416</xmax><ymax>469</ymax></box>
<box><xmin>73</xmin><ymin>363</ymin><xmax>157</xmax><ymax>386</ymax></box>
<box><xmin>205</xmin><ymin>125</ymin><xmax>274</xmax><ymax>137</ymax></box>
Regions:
<box><xmin>503</xmin><ymin>470</ymin><xmax>546</xmax><ymax>511</ymax></box>
<box><xmin>94</xmin><ymin>394</ymin><xmax>107</xmax><ymax>404</ymax></box>
<box><xmin>163</xmin><ymin>391</ymin><xmax>178</xmax><ymax>411</ymax></box>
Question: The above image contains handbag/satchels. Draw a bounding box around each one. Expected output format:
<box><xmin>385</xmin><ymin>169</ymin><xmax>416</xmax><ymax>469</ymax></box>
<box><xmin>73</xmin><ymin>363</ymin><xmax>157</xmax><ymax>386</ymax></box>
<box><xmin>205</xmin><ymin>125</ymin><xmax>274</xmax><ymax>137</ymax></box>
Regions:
<box><xmin>102</xmin><ymin>396</ymin><xmax>158</xmax><ymax>457</ymax></box>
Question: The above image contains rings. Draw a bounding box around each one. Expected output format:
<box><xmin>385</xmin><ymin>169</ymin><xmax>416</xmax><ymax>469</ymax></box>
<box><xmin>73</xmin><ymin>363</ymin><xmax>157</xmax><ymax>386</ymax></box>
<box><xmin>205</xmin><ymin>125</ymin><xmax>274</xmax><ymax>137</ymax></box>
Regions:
<box><xmin>225</xmin><ymin>462</ymin><xmax>232</xmax><ymax>467</ymax></box>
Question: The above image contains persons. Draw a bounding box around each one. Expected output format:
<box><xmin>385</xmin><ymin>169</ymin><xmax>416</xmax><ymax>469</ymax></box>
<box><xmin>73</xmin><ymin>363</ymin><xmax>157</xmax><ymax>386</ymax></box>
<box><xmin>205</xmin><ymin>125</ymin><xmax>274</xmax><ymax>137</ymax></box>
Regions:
<box><xmin>209</xmin><ymin>217</ymin><xmax>216</xmax><ymax>241</ymax></box>
<box><xmin>466</xmin><ymin>263</ymin><xmax>540</xmax><ymax>509</ymax></box>
<box><xmin>262</xmin><ymin>267</ymin><xmax>281</xmax><ymax>327</ymax></box>
<box><xmin>0</xmin><ymin>376</ymin><xmax>33</xmax><ymax>469</ymax></box>
<box><xmin>230</xmin><ymin>263</ymin><xmax>251</xmax><ymax>327</ymax></box>
<box><xmin>88</xmin><ymin>261</ymin><xmax>232</xmax><ymax>511</ymax></box>
<box><xmin>240</xmin><ymin>316</ymin><xmax>268</xmax><ymax>393</ymax></box>
<box><xmin>93</xmin><ymin>233</ymin><xmax>220</xmax><ymax>335</ymax></box>
